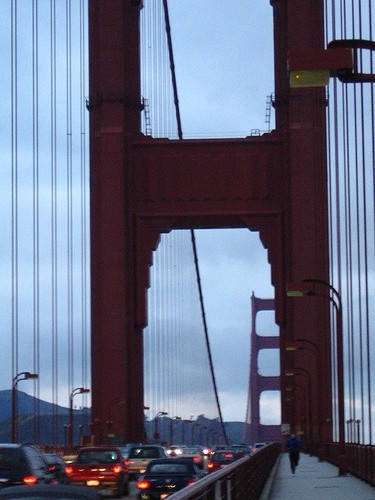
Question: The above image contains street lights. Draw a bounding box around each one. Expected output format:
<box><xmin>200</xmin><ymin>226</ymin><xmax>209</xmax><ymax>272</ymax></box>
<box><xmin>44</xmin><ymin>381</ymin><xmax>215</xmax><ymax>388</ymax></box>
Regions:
<box><xmin>12</xmin><ymin>372</ymin><xmax>39</xmax><ymax>444</ymax></box>
<box><xmin>155</xmin><ymin>412</ymin><xmax>222</xmax><ymax>448</ymax></box>
<box><xmin>283</xmin><ymin>276</ymin><xmax>345</xmax><ymax>442</ymax></box>
<box><xmin>69</xmin><ymin>387</ymin><xmax>89</xmax><ymax>445</ymax></box>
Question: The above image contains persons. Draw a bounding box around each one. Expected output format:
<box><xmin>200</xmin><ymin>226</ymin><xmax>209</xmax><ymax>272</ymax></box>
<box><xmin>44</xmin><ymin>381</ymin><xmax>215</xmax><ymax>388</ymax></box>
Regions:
<box><xmin>286</xmin><ymin>433</ymin><xmax>302</xmax><ymax>477</ymax></box>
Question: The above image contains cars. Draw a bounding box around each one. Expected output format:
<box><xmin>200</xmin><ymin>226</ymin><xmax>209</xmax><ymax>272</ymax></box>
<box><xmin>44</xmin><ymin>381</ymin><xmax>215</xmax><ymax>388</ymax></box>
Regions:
<box><xmin>0</xmin><ymin>442</ymin><xmax>268</xmax><ymax>500</ymax></box>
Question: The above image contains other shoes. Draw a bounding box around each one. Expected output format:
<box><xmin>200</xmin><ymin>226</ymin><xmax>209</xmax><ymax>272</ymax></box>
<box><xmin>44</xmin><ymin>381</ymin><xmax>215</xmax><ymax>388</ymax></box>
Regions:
<box><xmin>292</xmin><ymin>474</ymin><xmax>296</xmax><ymax>477</ymax></box>
